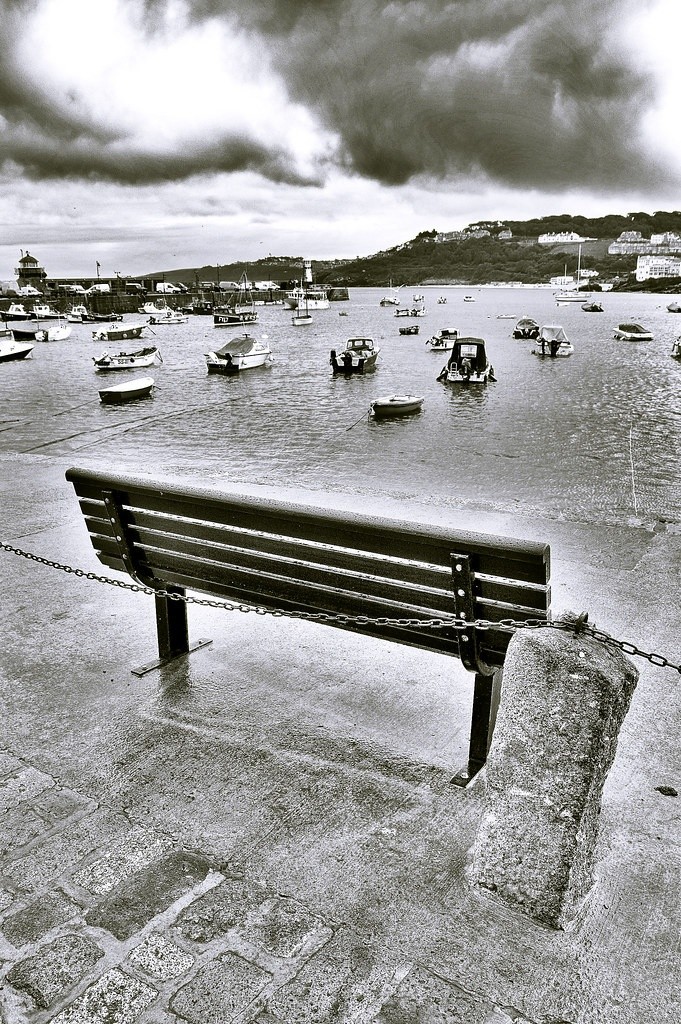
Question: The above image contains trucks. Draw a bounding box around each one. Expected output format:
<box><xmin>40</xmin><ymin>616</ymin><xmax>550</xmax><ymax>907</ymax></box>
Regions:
<box><xmin>172</xmin><ymin>282</ymin><xmax>188</xmax><ymax>293</ymax></box>
<box><xmin>2</xmin><ymin>282</ymin><xmax>43</xmax><ymax>298</ymax></box>
<box><xmin>156</xmin><ymin>282</ymin><xmax>181</xmax><ymax>294</ymax></box>
<box><xmin>59</xmin><ymin>285</ymin><xmax>110</xmax><ymax>296</ymax></box>
<box><xmin>219</xmin><ymin>281</ymin><xmax>281</xmax><ymax>292</ymax></box>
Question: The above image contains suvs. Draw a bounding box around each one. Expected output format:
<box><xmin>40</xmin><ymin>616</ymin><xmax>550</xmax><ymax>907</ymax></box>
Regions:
<box><xmin>126</xmin><ymin>284</ymin><xmax>147</xmax><ymax>295</ymax></box>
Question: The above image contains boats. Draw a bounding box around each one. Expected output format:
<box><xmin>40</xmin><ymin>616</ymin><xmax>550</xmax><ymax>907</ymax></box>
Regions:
<box><xmin>580</xmin><ymin>301</ymin><xmax>605</xmax><ymax>312</ymax></box>
<box><xmin>92</xmin><ymin>346</ymin><xmax>163</xmax><ymax>370</ymax></box>
<box><xmin>464</xmin><ymin>295</ymin><xmax>475</xmax><ymax>302</ymax></box>
<box><xmin>425</xmin><ymin>328</ymin><xmax>497</xmax><ymax>387</ymax></box>
<box><xmin>667</xmin><ymin>301</ymin><xmax>681</xmax><ymax>313</ymax></box>
<box><xmin>437</xmin><ymin>296</ymin><xmax>448</xmax><ymax>304</ymax></box>
<box><xmin>497</xmin><ymin>314</ymin><xmax>516</xmax><ymax>319</ymax></box>
<box><xmin>512</xmin><ymin>314</ymin><xmax>575</xmax><ymax>358</ymax></box>
<box><xmin>670</xmin><ymin>335</ymin><xmax>681</xmax><ymax>362</ymax></box>
<box><xmin>0</xmin><ymin>301</ymin><xmax>124</xmax><ymax>363</ymax></box>
<box><xmin>613</xmin><ymin>323</ymin><xmax>654</xmax><ymax>342</ymax></box>
<box><xmin>203</xmin><ymin>321</ymin><xmax>273</xmax><ymax>374</ymax></box>
<box><xmin>379</xmin><ymin>292</ymin><xmax>428</xmax><ymax>317</ymax></box>
<box><xmin>92</xmin><ymin>322</ymin><xmax>150</xmax><ymax>341</ymax></box>
<box><xmin>98</xmin><ymin>377</ymin><xmax>155</xmax><ymax>404</ymax></box>
<box><xmin>329</xmin><ymin>338</ymin><xmax>382</xmax><ymax>379</ymax></box>
<box><xmin>280</xmin><ymin>277</ymin><xmax>331</xmax><ymax>326</ymax></box>
<box><xmin>399</xmin><ymin>324</ymin><xmax>420</xmax><ymax>335</ymax></box>
<box><xmin>370</xmin><ymin>394</ymin><xmax>424</xmax><ymax>418</ymax></box>
<box><xmin>137</xmin><ymin>271</ymin><xmax>260</xmax><ymax>326</ymax></box>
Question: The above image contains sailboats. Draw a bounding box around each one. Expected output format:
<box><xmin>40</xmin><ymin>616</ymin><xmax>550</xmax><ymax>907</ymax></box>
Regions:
<box><xmin>553</xmin><ymin>243</ymin><xmax>591</xmax><ymax>303</ymax></box>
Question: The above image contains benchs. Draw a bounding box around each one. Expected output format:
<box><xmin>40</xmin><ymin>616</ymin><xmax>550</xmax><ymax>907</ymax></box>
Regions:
<box><xmin>65</xmin><ymin>467</ymin><xmax>551</xmax><ymax>788</ymax></box>
